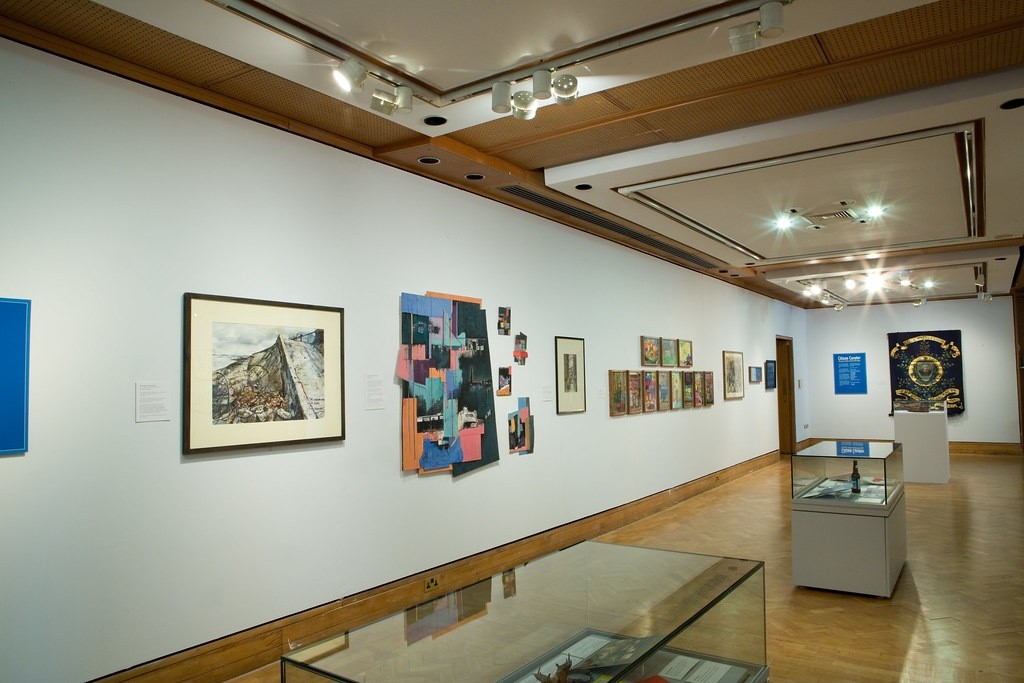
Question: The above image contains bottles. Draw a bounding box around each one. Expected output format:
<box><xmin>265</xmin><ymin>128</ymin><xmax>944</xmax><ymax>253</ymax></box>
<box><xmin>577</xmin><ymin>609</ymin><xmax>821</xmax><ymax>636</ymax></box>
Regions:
<box><xmin>849</xmin><ymin>460</ymin><xmax>861</xmax><ymax>493</ymax></box>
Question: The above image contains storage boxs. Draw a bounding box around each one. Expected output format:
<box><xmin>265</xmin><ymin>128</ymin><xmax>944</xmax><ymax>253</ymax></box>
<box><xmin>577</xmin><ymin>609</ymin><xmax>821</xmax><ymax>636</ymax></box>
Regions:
<box><xmin>791</xmin><ymin>440</ymin><xmax>904</xmax><ymax>506</ymax></box>
<box><xmin>280</xmin><ymin>538</ymin><xmax>768</xmax><ymax>683</ymax></box>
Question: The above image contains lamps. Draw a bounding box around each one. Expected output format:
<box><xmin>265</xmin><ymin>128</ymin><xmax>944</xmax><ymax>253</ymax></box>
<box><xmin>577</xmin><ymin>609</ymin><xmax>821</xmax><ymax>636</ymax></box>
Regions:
<box><xmin>820</xmin><ymin>279</ymin><xmax>827</xmax><ymax>289</ymax></box>
<box><xmin>821</xmin><ymin>293</ymin><xmax>830</xmax><ymax>304</ymax></box>
<box><xmin>899</xmin><ymin>270</ymin><xmax>911</xmax><ymax>286</ymax></box>
<box><xmin>333</xmin><ymin>57</ymin><xmax>368</xmax><ymax>93</ymax></box>
<box><xmin>834</xmin><ymin>300</ymin><xmax>843</xmax><ymax>311</ymax></box>
<box><xmin>913</xmin><ymin>300</ymin><xmax>922</xmax><ymax>307</ymax></box>
<box><xmin>984</xmin><ymin>294</ymin><xmax>992</xmax><ymax>304</ymax></box>
<box><xmin>759</xmin><ymin>2</ymin><xmax>784</xmax><ymax>40</ymax></box>
<box><xmin>491</xmin><ymin>83</ymin><xmax>512</xmax><ymax>113</ymax></box>
<box><xmin>532</xmin><ymin>71</ymin><xmax>552</xmax><ymax>101</ymax></box>
<box><xmin>973</xmin><ymin>274</ymin><xmax>984</xmax><ymax>286</ymax></box>
<box><xmin>978</xmin><ymin>292</ymin><xmax>985</xmax><ymax>303</ymax></box>
<box><xmin>728</xmin><ymin>21</ymin><xmax>761</xmax><ymax>53</ymax></box>
<box><xmin>553</xmin><ymin>73</ymin><xmax>578</xmax><ymax>107</ymax></box>
<box><xmin>511</xmin><ymin>91</ymin><xmax>538</xmax><ymax>121</ymax></box>
<box><xmin>803</xmin><ymin>284</ymin><xmax>813</xmax><ymax>296</ymax></box>
<box><xmin>369</xmin><ymin>88</ymin><xmax>399</xmax><ymax>116</ymax></box>
<box><xmin>393</xmin><ymin>86</ymin><xmax>413</xmax><ymax>115</ymax></box>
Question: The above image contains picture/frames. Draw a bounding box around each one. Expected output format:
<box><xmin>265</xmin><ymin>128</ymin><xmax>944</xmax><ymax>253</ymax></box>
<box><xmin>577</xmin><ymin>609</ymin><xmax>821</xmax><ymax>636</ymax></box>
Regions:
<box><xmin>683</xmin><ymin>371</ymin><xmax>693</xmax><ymax>408</ymax></box>
<box><xmin>671</xmin><ymin>371</ymin><xmax>683</xmax><ymax>410</ymax></box>
<box><xmin>658</xmin><ymin>370</ymin><xmax>671</xmax><ymax>411</ymax></box>
<box><xmin>608</xmin><ymin>370</ymin><xmax>627</xmax><ymax>417</ymax></box>
<box><xmin>723</xmin><ymin>351</ymin><xmax>745</xmax><ymax>401</ymax></box>
<box><xmin>693</xmin><ymin>371</ymin><xmax>704</xmax><ymax>407</ymax></box>
<box><xmin>677</xmin><ymin>339</ymin><xmax>694</xmax><ymax>368</ymax></box>
<box><xmin>765</xmin><ymin>359</ymin><xmax>777</xmax><ymax>389</ymax></box>
<box><xmin>640</xmin><ymin>335</ymin><xmax>661</xmax><ymax>366</ymax></box>
<box><xmin>643</xmin><ymin>370</ymin><xmax>657</xmax><ymax>413</ymax></box>
<box><xmin>661</xmin><ymin>338</ymin><xmax>677</xmax><ymax>367</ymax></box>
<box><xmin>704</xmin><ymin>371</ymin><xmax>715</xmax><ymax>406</ymax></box>
<box><xmin>183</xmin><ymin>293</ymin><xmax>345</xmax><ymax>455</ymax></box>
<box><xmin>555</xmin><ymin>336</ymin><xmax>588</xmax><ymax>414</ymax></box>
<box><xmin>627</xmin><ymin>370</ymin><xmax>643</xmax><ymax>415</ymax></box>
